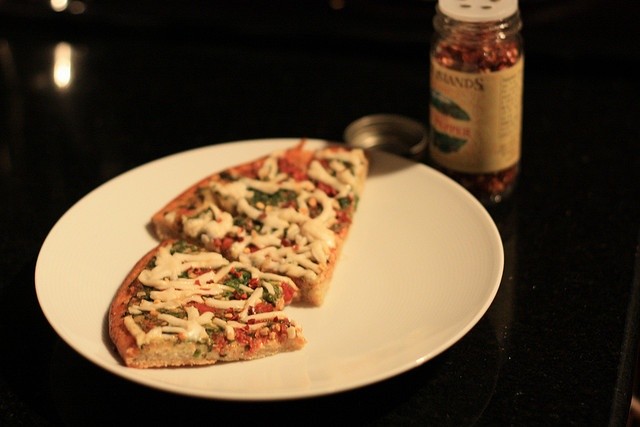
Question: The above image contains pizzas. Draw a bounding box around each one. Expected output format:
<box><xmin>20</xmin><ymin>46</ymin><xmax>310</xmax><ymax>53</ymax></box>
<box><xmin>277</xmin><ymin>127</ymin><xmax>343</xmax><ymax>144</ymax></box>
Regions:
<box><xmin>151</xmin><ymin>146</ymin><xmax>370</xmax><ymax>307</ymax></box>
<box><xmin>106</xmin><ymin>239</ymin><xmax>307</xmax><ymax>369</ymax></box>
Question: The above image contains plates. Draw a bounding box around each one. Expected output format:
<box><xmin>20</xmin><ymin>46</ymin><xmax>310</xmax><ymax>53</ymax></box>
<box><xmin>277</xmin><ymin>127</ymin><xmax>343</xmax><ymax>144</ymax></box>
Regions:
<box><xmin>34</xmin><ymin>138</ymin><xmax>506</xmax><ymax>401</ymax></box>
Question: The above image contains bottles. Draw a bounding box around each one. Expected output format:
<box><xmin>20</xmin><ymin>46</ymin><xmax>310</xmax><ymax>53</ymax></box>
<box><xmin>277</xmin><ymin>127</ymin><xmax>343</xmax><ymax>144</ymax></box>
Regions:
<box><xmin>427</xmin><ymin>0</ymin><xmax>526</xmax><ymax>203</ymax></box>
